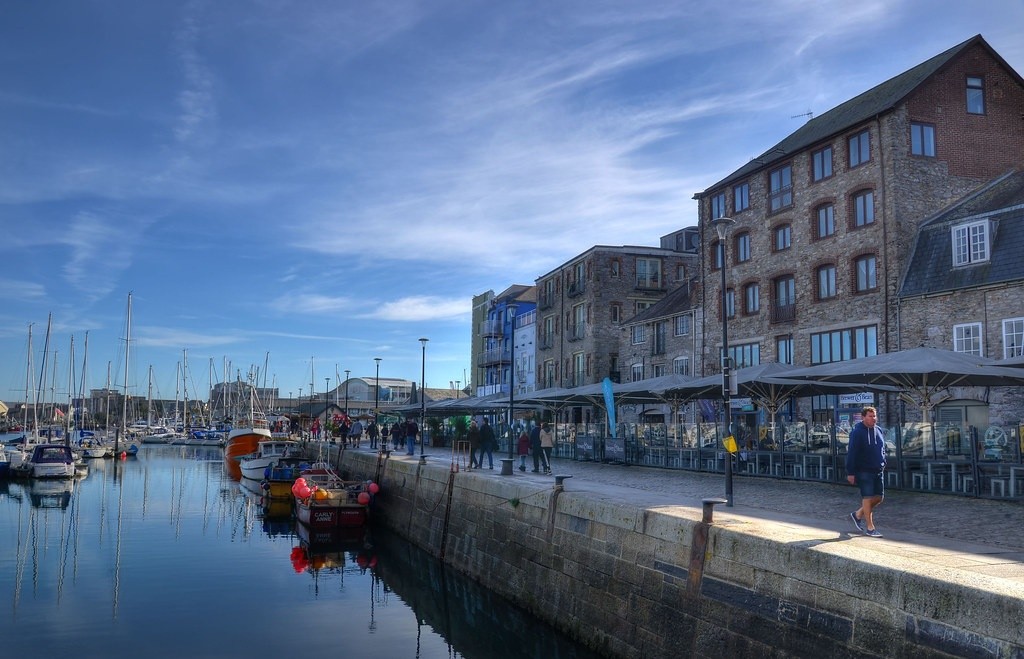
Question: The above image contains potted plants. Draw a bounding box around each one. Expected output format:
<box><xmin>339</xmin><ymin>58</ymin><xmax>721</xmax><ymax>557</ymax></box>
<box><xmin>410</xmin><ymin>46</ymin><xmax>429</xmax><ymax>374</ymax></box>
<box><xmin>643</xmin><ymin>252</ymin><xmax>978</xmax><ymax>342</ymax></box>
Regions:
<box><xmin>426</xmin><ymin>418</ymin><xmax>444</xmax><ymax>447</ymax></box>
<box><xmin>451</xmin><ymin>416</ymin><xmax>469</xmax><ymax>449</ymax></box>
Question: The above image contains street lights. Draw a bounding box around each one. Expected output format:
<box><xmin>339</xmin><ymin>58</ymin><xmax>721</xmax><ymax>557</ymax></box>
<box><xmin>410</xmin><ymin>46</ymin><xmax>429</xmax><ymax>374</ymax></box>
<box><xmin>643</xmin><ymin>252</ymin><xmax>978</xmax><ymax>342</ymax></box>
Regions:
<box><xmin>507</xmin><ymin>303</ymin><xmax>520</xmax><ymax>459</ymax></box>
<box><xmin>374</xmin><ymin>357</ymin><xmax>382</xmax><ymax>447</ymax></box>
<box><xmin>345</xmin><ymin>370</ymin><xmax>352</xmax><ymax>426</ymax></box>
<box><xmin>324</xmin><ymin>377</ymin><xmax>332</xmax><ymax>440</ymax></box>
<box><xmin>290</xmin><ymin>392</ymin><xmax>293</xmax><ymax>421</ymax></box>
<box><xmin>308</xmin><ymin>383</ymin><xmax>314</xmax><ymax>430</ymax></box>
<box><xmin>298</xmin><ymin>388</ymin><xmax>303</xmax><ymax>435</ymax></box>
<box><xmin>419</xmin><ymin>338</ymin><xmax>429</xmax><ymax>454</ymax></box>
<box><xmin>708</xmin><ymin>217</ymin><xmax>736</xmax><ymax>502</ymax></box>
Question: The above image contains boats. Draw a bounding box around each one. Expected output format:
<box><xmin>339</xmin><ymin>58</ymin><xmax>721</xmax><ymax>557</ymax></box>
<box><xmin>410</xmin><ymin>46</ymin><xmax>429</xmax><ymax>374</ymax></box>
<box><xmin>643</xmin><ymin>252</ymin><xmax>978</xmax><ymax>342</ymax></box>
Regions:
<box><xmin>294</xmin><ymin>439</ymin><xmax>374</xmax><ymax>529</ymax></box>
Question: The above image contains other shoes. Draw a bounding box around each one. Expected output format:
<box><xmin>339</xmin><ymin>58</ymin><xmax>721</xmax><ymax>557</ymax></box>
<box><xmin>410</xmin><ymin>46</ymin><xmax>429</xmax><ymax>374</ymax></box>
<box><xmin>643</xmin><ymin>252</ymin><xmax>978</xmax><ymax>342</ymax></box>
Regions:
<box><xmin>531</xmin><ymin>469</ymin><xmax>539</xmax><ymax>472</ymax></box>
<box><xmin>543</xmin><ymin>471</ymin><xmax>547</xmax><ymax>474</ymax></box>
<box><xmin>866</xmin><ymin>528</ymin><xmax>883</xmax><ymax>537</ymax></box>
<box><xmin>479</xmin><ymin>466</ymin><xmax>482</xmax><ymax>469</ymax></box>
<box><xmin>489</xmin><ymin>467</ymin><xmax>493</xmax><ymax>469</ymax></box>
<box><xmin>546</xmin><ymin>471</ymin><xmax>552</xmax><ymax>475</ymax></box>
<box><xmin>519</xmin><ymin>467</ymin><xmax>525</xmax><ymax>472</ymax></box>
<box><xmin>467</xmin><ymin>465</ymin><xmax>472</xmax><ymax>468</ymax></box>
<box><xmin>851</xmin><ymin>512</ymin><xmax>865</xmax><ymax>531</ymax></box>
<box><xmin>474</xmin><ymin>465</ymin><xmax>479</xmax><ymax>469</ymax></box>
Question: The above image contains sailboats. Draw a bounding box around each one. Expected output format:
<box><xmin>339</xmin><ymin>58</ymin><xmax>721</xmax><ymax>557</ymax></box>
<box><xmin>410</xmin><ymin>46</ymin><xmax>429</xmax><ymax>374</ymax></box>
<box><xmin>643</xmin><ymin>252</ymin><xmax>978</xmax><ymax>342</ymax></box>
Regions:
<box><xmin>0</xmin><ymin>285</ymin><xmax>334</xmax><ymax>484</ymax></box>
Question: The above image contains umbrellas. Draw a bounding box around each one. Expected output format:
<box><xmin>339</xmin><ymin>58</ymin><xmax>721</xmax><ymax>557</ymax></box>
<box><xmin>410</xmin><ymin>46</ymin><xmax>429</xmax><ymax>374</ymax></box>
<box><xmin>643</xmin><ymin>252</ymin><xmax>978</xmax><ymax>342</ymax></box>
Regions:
<box><xmin>449</xmin><ymin>391</ymin><xmax>535</xmax><ymax>435</ymax></box>
<box><xmin>577</xmin><ymin>372</ymin><xmax>702</xmax><ymax>447</ymax></box>
<box><xmin>528</xmin><ymin>380</ymin><xmax>624</xmax><ymax>438</ymax></box>
<box><xmin>978</xmin><ymin>353</ymin><xmax>1024</xmax><ymax>369</ymax></box>
<box><xmin>410</xmin><ymin>382</ymin><xmax>419</xmax><ymax>422</ymax></box>
<box><xmin>648</xmin><ymin>361</ymin><xmax>910</xmax><ymax>451</ymax></box>
<box><xmin>758</xmin><ymin>344</ymin><xmax>1024</xmax><ymax>456</ymax></box>
<box><xmin>391</xmin><ymin>397</ymin><xmax>478</xmax><ymax>430</ymax></box>
<box><xmin>486</xmin><ymin>387</ymin><xmax>592</xmax><ymax>440</ymax></box>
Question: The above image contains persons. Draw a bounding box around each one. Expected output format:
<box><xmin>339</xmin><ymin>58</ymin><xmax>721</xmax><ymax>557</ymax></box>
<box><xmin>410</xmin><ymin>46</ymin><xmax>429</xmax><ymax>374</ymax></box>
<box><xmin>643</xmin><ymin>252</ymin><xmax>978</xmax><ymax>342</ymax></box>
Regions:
<box><xmin>846</xmin><ymin>407</ymin><xmax>886</xmax><ymax>537</ymax></box>
<box><xmin>529</xmin><ymin>422</ymin><xmax>547</xmax><ymax>473</ymax></box>
<box><xmin>338</xmin><ymin>418</ymin><xmax>363</xmax><ymax>449</ymax></box>
<box><xmin>405</xmin><ymin>420</ymin><xmax>418</xmax><ymax>455</ymax></box>
<box><xmin>389</xmin><ymin>422</ymin><xmax>406</xmax><ymax>451</ymax></box>
<box><xmin>539</xmin><ymin>422</ymin><xmax>555</xmax><ymax>474</ymax></box>
<box><xmin>381</xmin><ymin>423</ymin><xmax>389</xmax><ymax>444</ymax></box>
<box><xmin>466</xmin><ymin>421</ymin><xmax>478</xmax><ymax>469</ymax></box>
<box><xmin>365</xmin><ymin>421</ymin><xmax>379</xmax><ymax>449</ymax></box>
<box><xmin>478</xmin><ymin>417</ymin><xmax>495</xmax><ymax>469</ymax></box>
<box><xmin>310</xmin><ymin>421</ymin><xmax>322</xmax><ymax>440</ymax></box>
<box><xmin>517</xmin><ymin>431</ymin><xmax>531</xmax><ymax>471</ymax></box>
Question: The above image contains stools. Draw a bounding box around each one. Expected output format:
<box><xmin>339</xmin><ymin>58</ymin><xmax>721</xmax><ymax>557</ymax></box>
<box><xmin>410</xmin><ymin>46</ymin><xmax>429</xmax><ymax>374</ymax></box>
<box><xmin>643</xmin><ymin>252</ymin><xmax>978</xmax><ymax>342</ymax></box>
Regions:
<box><xmin>552</xmin><ymin>448</ymin><xmax>574</xmax><ymax>457</ymax></box>
<box><xmin>643</xmin><ymin>455</ymin><xmax>1005</xmax><ymax>497</ymax></box>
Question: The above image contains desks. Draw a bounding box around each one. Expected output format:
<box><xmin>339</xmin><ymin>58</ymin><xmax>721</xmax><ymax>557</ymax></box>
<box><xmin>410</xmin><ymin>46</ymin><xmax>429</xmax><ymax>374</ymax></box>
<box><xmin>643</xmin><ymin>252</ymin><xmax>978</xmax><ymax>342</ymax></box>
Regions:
<box><xmin>563</xmin><ymin>443</ymin><xmax>571</xmax><ymax>457</ymax></box>
<box><xmin>647</xmin><ymin>446</ymin><xmax>1017</xmax><ymax>497</ymax></box>
